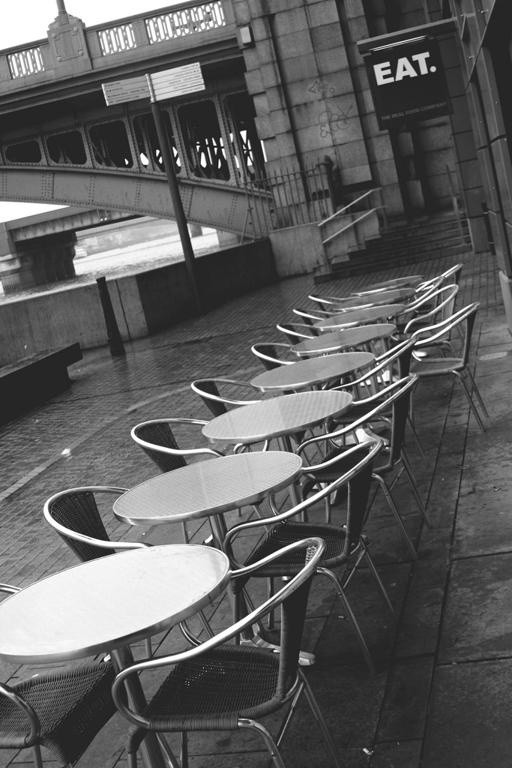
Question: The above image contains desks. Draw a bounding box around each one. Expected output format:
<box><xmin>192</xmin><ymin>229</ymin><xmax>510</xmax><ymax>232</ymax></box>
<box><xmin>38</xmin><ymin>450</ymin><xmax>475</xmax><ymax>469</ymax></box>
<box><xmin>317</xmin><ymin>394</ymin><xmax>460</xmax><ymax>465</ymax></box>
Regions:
<box><xmin>197</xmin><ymin>387</ymin><xmax>374</xmax><ymax>551</ymax></box>
<box><xmin>3</xmin><ymin>542</ymin><xmax>236</xmax><ymax>768</ymax></box>
<box><xmin>110</xmin><ymin>446</ymin><xmax>332</xmax><ymax>670</ymax></box>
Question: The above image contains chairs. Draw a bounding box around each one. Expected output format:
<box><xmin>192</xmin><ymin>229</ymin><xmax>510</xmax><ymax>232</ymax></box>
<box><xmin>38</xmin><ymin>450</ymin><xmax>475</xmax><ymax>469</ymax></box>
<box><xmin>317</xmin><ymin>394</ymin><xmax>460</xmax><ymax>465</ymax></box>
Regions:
<box><xmin>36</xmin><ymin>483</ymin><xmax>219</xmax><ymax>671</ymax></box>
<box><xmin>0</xmin><ymin>581</ymin><xmax>181</xmax><ymax>766</ymax></box>
<box><xmin>106</xmin><ymin>535</ymin><xmax>344</xmax><ymax>768</ymax></box>
<box><xmin>128</xmin><ymin>414</ymin><xmax>279</xmax><ymax>543</ymax></box>
<box><xmin>320</xmin><ymin>371</ymin><xmax>436</xmax><ymax>564</ymax></box>
<box><xmin>221</xmin><ymin>437</ymin><xmax>402</xmax><ymax>678</ymax></box>
<box><xmin>243</xmin><ymin>265</ymin><xmax>486</xmax><ymax>458</ymax></box>
